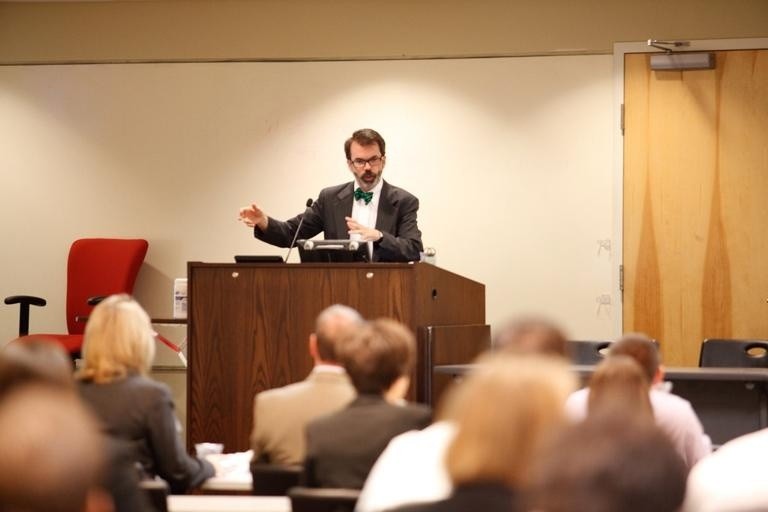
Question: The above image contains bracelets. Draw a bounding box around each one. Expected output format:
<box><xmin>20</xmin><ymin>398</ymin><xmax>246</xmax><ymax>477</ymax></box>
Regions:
<box><xmin>376</xmin><ymin>229</ymin><xmax>383</xmax><ymax>242</ymax></box>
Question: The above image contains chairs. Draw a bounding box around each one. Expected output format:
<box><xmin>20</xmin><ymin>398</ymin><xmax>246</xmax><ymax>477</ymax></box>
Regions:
<box><xmin>562</xmin><ymin>339</ymin><xmax>613</xmax><ymax>366</ymax></box>
<box><xmin>4</xmin><ymin>239</ymin><xmax>148</xmax><ymax>362</ymax></box>
<box><xmin>289</xmin><ymin>486</ymin><xmax>361</xmax><ymax>511</ymax></box>
<box><xmin>699</xmin><ymin>337</ymin><xmax>768</xmax><ymax>367</ymax></box>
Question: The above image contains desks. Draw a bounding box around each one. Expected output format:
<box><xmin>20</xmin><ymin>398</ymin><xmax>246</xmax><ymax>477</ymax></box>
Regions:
<box><xmin>434</xmin><ymin>363</ymin><xmax>768</xmax><ymax>451</ymax></box>
<box><xmin>138</xmin><ymin>449</ymin><xmax>254</xmax><ymax>512</ymax></box>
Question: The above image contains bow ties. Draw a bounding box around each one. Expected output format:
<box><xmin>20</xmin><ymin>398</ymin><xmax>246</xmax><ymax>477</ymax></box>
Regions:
<box><xmin>354</xmin><ymin>187</ymin><xmax>374</xmax><ymax>205</ymax></box>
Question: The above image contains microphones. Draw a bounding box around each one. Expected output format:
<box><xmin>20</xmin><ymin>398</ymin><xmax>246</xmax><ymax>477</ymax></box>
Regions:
<box><xmin>284</xmin><ymin>197</ymin><xmax>314</xmax><ymax>263</ymax></box>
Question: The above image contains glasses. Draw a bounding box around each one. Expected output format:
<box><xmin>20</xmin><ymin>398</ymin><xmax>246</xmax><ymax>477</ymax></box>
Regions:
<box><xmin>352</xmin><ymin>155</ymin><xmax>383</xmax><ymax>169</ymax></box>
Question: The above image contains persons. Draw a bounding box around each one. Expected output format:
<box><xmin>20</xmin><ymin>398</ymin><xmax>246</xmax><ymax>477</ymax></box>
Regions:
<box><xmin>1</xmin><ymin>292</ymin><xmax>218</xmax><ymax>511</ymax></box>
<box><xmin>240</xmin><ymin>127</ymin><xmax>427</xmax><ymax>266</ymax></box>
<box><xmin>251</xmin><ymin>303</ymin><xmax>767</xmax><ymax>512</ymax></box>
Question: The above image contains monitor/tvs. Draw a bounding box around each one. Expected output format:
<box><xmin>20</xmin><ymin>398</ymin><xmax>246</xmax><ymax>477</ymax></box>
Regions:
<box><xmin>295</xmin><ymin>237</ymin><xmax>372</xmax><ymax>263</ymax></box>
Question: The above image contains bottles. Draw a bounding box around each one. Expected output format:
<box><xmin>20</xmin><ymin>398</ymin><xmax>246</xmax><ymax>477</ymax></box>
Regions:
<box><xmin>425</xmin><ymin>247</ymin><xmax>436</xmax><ymax>267</ymax></box>
